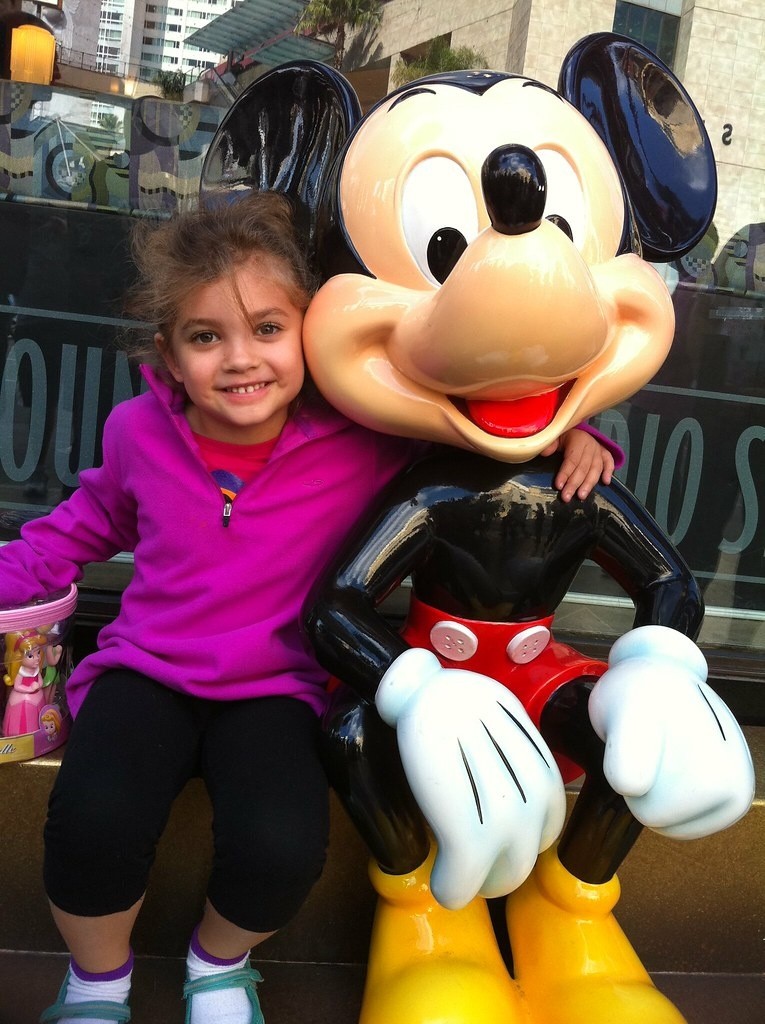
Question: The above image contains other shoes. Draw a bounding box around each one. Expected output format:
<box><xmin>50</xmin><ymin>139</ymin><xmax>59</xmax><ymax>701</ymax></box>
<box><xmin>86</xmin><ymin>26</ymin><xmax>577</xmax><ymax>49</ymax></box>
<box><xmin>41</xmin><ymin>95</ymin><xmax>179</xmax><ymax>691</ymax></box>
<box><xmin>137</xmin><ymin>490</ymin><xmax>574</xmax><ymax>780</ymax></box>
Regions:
<box><xmin>40</xmin><ymin>967</ymin><xmax>131</xmax><ymax>1024</ymax></box>
<box><xmin>182</xmin><ymin>956</ymin><xmax>264</xmax><ymax>1024</ymax></box>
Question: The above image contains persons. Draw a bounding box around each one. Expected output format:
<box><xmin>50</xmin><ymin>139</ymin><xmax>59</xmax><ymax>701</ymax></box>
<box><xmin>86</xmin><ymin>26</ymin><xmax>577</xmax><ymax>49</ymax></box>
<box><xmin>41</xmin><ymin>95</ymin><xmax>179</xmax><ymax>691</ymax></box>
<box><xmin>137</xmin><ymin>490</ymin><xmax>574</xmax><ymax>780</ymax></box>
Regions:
<box><xmin>0</xmin><ymin>193</ymin><xmax>628</xmax><ymax>1024</ymax></box>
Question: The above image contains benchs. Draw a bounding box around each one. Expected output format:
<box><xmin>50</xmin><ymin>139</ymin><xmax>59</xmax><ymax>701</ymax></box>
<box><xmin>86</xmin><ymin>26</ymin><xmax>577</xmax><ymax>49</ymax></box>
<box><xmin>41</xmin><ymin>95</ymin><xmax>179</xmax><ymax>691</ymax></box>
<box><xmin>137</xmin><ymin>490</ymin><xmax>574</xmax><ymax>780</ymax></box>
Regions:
<box><xmin>0</xmin><ymin>727</ymin><xmax>764</xmax><ymax>976</ymax></box>
